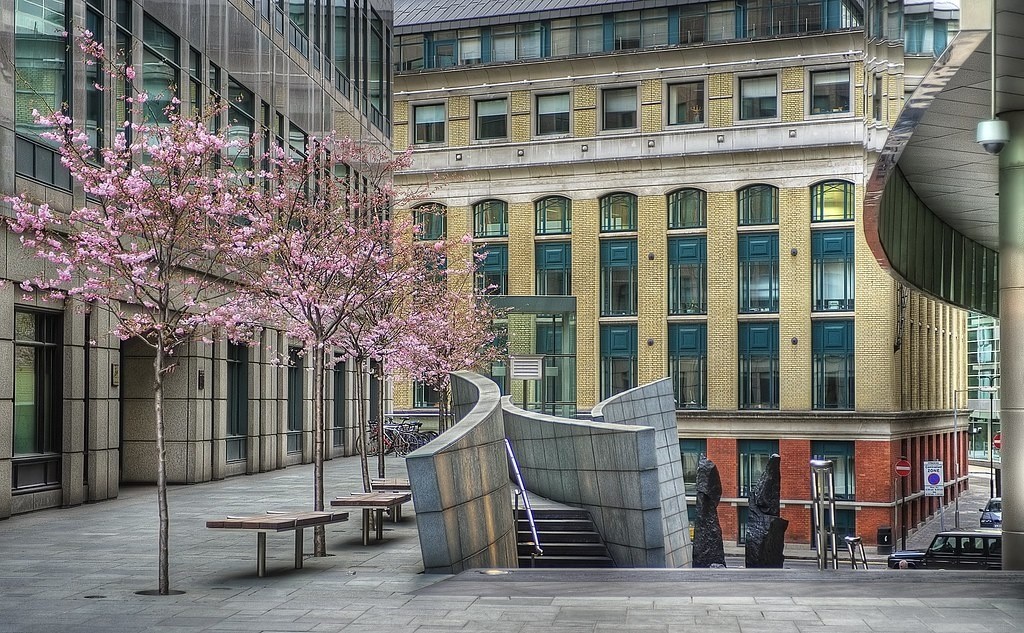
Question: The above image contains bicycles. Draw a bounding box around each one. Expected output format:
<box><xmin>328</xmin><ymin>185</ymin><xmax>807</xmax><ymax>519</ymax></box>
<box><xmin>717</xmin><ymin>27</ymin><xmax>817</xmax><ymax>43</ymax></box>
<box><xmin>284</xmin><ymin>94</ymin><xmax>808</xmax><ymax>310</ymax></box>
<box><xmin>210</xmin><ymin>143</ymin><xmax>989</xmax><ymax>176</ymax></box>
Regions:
<box><xmin>356</xmin><ymin>414</ymin><xmax>440</xmax><ymax>458</ymax></box>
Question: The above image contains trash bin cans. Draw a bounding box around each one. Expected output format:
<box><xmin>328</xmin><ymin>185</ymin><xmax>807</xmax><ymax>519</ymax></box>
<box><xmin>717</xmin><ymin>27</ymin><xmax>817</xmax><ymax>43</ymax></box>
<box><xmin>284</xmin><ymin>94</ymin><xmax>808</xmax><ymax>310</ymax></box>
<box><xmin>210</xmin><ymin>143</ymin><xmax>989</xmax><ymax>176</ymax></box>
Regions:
<box><xmin>877</xmin><ymin>526</ymin><xmax>893</xmax><ymax>555</ymax></box>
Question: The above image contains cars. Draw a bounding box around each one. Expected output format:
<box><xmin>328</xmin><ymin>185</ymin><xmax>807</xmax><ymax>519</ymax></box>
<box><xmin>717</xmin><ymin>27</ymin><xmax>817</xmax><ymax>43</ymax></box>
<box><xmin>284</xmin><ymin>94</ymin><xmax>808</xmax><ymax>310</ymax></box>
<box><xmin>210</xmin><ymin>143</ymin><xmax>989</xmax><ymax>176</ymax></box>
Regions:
<box><xmin>978</xmin><ymin>498</ymin><xmax>1002</xmax><ymax>528</ymax></box>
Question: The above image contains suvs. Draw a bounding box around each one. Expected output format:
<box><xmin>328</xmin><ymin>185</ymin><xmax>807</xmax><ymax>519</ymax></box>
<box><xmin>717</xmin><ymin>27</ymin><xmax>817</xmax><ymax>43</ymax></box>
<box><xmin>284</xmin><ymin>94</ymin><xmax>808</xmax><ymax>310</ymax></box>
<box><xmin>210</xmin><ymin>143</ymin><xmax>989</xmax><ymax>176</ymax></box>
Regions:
<box><xmin>887</xmin><ymin>530</ymin><xmax>1002</xmax><ymax>570</ymax></box>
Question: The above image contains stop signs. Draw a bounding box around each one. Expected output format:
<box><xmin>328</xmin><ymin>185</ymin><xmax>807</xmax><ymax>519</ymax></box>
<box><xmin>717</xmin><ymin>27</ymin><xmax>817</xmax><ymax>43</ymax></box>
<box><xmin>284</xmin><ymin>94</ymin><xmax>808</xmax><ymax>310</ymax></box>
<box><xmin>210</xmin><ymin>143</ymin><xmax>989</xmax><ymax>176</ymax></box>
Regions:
<box><xmin>993</xmin><ymin>434</ymin><xmax>1000</xmax><ymax>448</ymax></box>
<box><xmin>894</xmin><ymin>460</ymin><xmax>912</xmax><ymax>477</ymax></box>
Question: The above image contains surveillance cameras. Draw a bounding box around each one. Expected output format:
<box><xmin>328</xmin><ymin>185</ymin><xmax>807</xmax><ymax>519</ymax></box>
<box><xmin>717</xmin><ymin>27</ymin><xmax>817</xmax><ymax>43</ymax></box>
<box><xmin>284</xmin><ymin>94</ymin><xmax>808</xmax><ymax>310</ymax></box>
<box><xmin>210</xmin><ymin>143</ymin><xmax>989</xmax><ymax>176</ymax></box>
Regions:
<box><xmin>977</xmin><ymin>120</ymin><xmax>1011</xmax><ymax>154</ymax></box>
